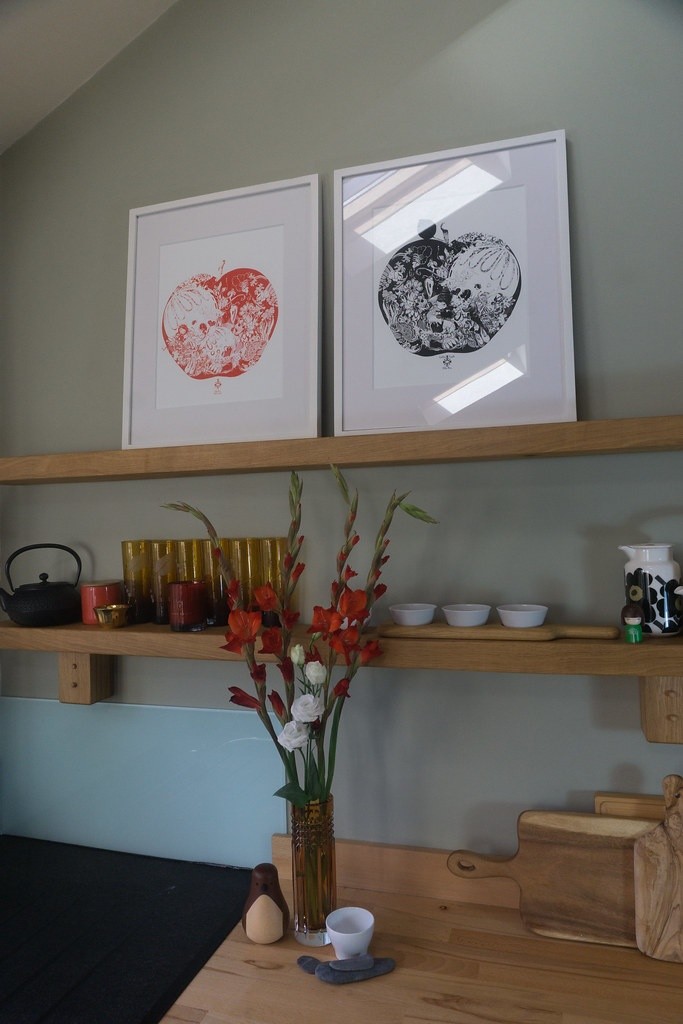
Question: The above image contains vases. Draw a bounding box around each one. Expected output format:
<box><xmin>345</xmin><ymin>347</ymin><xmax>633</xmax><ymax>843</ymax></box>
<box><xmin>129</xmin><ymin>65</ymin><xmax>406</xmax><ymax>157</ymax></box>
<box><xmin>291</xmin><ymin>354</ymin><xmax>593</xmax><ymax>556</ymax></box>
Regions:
<box><xmin>287</xmin><ymin>793</ymin><xmax>339</xmax><ymax>949</ymax></box>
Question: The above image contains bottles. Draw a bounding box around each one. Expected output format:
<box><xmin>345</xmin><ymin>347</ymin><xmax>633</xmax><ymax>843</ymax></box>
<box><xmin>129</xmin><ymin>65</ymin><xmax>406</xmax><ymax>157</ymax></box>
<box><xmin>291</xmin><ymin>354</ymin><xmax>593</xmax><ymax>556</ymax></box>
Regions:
<box><xmin>618</xmin><ymin>542</ymin><xmax>680</xmax><ymax>636</ymax></box>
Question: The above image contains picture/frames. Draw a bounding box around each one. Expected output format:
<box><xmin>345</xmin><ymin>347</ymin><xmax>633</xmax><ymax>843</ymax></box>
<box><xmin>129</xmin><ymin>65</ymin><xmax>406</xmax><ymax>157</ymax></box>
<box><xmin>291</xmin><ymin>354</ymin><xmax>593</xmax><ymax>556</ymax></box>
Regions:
<box><xmin>120</xmin><ymin>171</ymin><xmax>325</xmax><ymax>453</ymax></box>
<box><xmin>332</xmin><ymin>127</ymin><xmax>579</xmax><ymax>438</ymax></box>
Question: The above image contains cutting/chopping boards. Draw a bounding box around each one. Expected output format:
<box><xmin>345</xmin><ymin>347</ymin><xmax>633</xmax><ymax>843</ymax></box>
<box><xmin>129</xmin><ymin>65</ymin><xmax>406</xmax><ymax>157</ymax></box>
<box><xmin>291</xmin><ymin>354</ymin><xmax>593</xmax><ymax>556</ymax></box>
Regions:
<box><xmin>447</xmin><ymin>807</ymin><xmax>666</xmax><ymax>948</ymax></box>
<box><xmin>595</xmin><ymin>791</ymin><xmax>668</xmax><ymax>821</ymax></box>
<box><xmin>634</xmin><ymin>773</ymin><xmax>683</xmax><ymax>965</ymax></box>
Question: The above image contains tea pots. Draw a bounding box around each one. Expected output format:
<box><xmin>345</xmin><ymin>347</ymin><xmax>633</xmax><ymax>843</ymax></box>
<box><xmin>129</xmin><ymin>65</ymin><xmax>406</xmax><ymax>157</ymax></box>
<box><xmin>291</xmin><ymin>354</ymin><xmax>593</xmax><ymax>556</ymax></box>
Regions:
<box><xmin>0</xmin><ymin>543</ymin><xmax>81</xmax><ymax>629</ymax></box>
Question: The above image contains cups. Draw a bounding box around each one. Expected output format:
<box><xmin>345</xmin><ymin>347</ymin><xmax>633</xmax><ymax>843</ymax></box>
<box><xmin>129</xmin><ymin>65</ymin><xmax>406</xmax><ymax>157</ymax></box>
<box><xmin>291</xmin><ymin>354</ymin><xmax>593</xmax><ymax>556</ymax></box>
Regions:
<box><xmin>229</xmin><ymin>538</ymin><xmax>258</xmax><ymax>614</ymax></box>
<box><xmin>120</xmin><ymin>540</ymin><xmax>152</xmax><ymax>623</ymax></box>
<box><xmin>203</xmin><ymin>538</ymin><xmax>231</xmax><ymax>627</ymax></box>
<box><xmin>80</xmin><ymin>578</ymin><xmax>123</xmax><ymax>625</ymax></box>
<box><xmin>326</xmin><ymin>907</ymin><xmax>374</xmax><ymax>960</ymax></box>
<box><xmin>170</xmin><ymin>580</ymin><xmax>206</xmax><ymax>631</ymax></box>
<box><xmin>177</xmin><ymin>540</ymin><xmax>205</xmax><ymax>582</ymax></box>
<box><xmin>150</xmin><ymin>539</ymin><xmax>172</xmax><ymax>625</ymax></box>
<box><xmin>260</xmin><ymin>536</ymin><xmax>293</xmax><ymax>628</ymax></box>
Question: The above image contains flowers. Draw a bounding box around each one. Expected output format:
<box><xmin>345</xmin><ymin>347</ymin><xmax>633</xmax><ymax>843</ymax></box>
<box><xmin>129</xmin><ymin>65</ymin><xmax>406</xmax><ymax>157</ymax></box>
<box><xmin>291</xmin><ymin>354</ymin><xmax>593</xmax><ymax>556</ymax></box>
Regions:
<box><xmin>160</xmin><ymin>458</ymin><xmax>443</xmax><ymax>808</ymax></box>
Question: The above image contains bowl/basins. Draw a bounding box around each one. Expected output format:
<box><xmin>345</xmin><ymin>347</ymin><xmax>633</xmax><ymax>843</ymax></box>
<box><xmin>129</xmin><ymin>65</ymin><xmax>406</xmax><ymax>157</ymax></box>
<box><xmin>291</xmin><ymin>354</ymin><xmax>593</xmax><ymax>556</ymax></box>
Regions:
<box><xmin>496</xmin><ymin>604</ymin><xmax>548</xmax><ymax>628</ymax></box>
<box><xmin>389</xmin><ymin>603</ymin><xmax>437</xmax><ymax>626</ymax></box>
<box><xmin>441</xmin><ymin>604</ymin><xmax>492</xmax><ymax>627</ymax></box>
<box><xmin>93</xmin><ymin>604</ymin><xmax>129</xmax><ymax>629</ymax></box>
